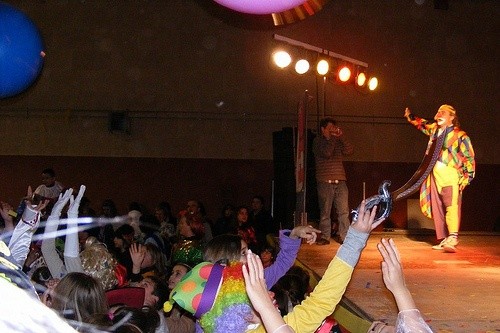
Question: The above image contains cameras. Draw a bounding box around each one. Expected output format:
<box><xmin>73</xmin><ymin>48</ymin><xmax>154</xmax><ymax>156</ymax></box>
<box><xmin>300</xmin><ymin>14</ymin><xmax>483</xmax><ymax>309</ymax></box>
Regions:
<box><xmin>335</xmin><ymin>130</ymin><xmax>340</xmax><ymax>135</ymax></box>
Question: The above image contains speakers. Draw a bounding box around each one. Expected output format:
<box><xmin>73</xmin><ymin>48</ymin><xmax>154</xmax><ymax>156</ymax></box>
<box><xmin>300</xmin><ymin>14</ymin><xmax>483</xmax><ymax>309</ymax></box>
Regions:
<box><xmin>273</xmin><ymin>126</ymin><xmax>320</xmax><ymax>237</ymax></box>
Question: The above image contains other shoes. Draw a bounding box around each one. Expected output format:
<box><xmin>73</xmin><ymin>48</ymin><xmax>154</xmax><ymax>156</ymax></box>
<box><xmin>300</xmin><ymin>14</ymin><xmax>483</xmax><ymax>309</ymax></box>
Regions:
<box><xmin>339</xmin><ymin>238</ymin><xmax>344</xmax><ymax>245</ymax></box>
<box><xmin>432</xmin><ymin>238</ymin><xmax>446</xmax><ymax>249</ymax></box>
<box><xmin>317</xmin><ymin>238</ymin><xmax>329</xmax><ymax>246</ymax></box>
<box><xmin>443</xmin><ymin>237</ymin><xmax>458</xmax><ymax>252</ymax></box>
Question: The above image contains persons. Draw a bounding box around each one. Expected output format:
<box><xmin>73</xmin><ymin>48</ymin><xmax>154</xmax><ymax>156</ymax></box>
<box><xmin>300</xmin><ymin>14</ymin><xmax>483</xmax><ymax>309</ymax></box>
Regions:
<box><xmin>390</xmin><ymin>104</ymin><xmax>476</xmax><ymax>253</ymax></box>
<box><xmin>0</xmin><ymin>168</ymin><xmax>434</xmax><ymax>333</ymax></box>
<box><xmin>312</xmin><ymin>118</ymin><xmax>356</xmax><ymax>245</ymax></box>
